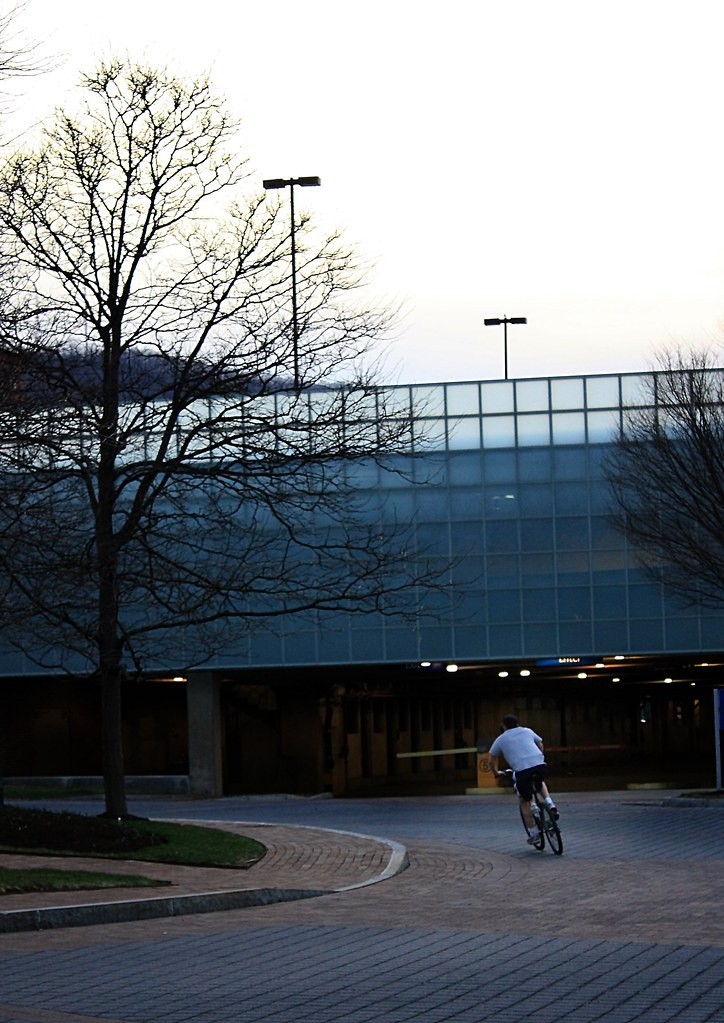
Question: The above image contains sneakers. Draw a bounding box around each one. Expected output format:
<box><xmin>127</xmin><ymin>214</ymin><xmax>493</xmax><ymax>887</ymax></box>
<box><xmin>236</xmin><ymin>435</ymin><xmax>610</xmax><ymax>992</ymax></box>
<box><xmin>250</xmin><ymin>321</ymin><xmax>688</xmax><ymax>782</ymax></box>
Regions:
<box><xmin>548</xmin><ymin>805</ymin><xmax>560</xmax><ymax>822</ymax></box>
<box><xmin>526</xmin><ymin>834</ymin><xmax>541</xmax><ymax>844</ymax></box>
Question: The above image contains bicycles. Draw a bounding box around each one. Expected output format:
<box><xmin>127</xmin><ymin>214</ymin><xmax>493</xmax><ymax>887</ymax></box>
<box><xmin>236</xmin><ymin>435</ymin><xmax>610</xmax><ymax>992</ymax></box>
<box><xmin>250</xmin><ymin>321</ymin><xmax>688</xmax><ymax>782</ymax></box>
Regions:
<box><xmin>495</xmin><ymin>769</ymin><xmax>563</xmax><ymax>855</ymax></box>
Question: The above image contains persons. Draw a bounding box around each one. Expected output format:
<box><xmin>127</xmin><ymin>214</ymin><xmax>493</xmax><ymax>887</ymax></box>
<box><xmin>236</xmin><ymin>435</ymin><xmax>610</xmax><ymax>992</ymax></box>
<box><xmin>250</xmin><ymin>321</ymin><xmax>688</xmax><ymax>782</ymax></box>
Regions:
<box><xmin>488</xmin><ymin>714</ymin><xmax>560</xmax><ymax>844</ymax></box>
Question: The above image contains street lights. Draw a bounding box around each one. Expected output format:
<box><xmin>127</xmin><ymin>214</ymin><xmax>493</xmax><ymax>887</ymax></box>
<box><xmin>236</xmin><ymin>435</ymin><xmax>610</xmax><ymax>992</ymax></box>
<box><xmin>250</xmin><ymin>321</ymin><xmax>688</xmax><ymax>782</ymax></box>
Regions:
<box><xmin>263</xmin><ymin>176</ymin><xmax>321</xmax><ymax>388</ymax></box>
<box><xmin>484</xmin><ymin>318</ymin><xmax>527</xmax><ymax>379</ymax></box>
